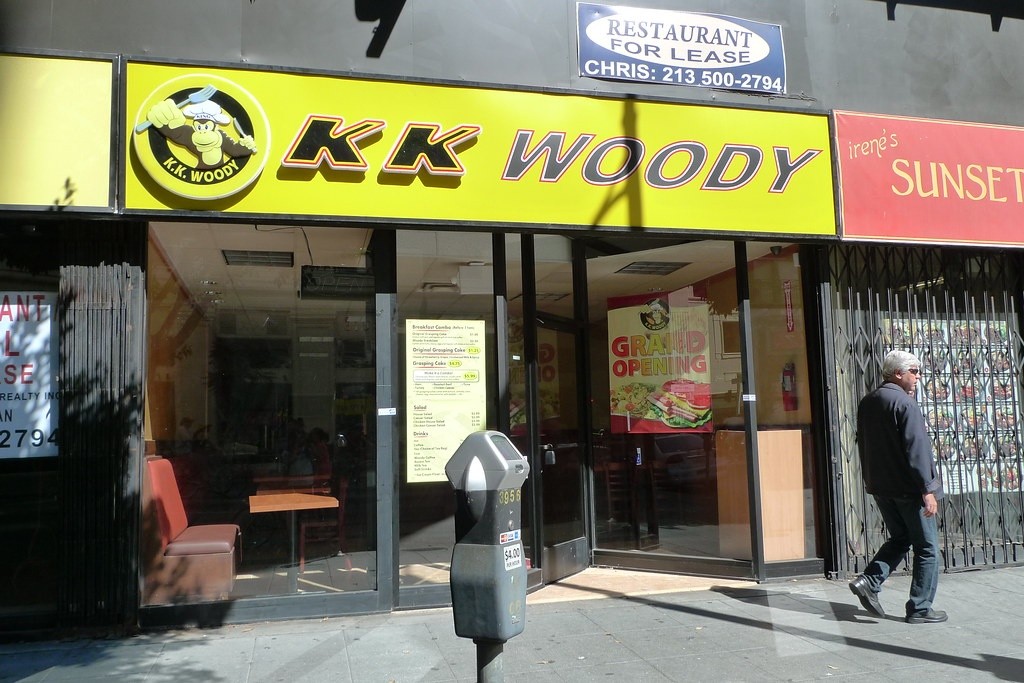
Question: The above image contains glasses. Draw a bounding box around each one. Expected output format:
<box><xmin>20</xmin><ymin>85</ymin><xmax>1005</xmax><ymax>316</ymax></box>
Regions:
<box><xmin>908</xmin><ymin>368</ymin><xmax>922</xmax><ymax>375</ymax></box>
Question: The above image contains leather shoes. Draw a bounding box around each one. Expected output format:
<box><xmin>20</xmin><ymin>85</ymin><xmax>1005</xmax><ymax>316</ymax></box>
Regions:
<box><xmin>849</xmin><ymin>574</ymin><xmax>885</xmax><ymax>618</ymax></box>
<box><xmin>905</xmin><ymin>602</ymin><xmax>948</xmax><ymax>624</ymax></box>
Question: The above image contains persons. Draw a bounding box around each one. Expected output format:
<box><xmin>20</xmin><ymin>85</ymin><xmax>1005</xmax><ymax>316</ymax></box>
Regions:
<box><xmin>176</xmin><ymin>417</ymin><xmax>195</xmax><ymax>456</ymax></box>
<box><xmin>849</xmin><ymin>350</ymin><xmax>949</xmax><ymax>623</ymax></box>
<box><xmin>193</xmin><ymin>415</ymin><xmax>330</xmax><ymax>502</ymax></box>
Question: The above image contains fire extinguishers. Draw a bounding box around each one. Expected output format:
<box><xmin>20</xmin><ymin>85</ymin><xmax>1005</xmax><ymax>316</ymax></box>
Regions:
<box><xmin>782</xmin><ymin>363</ymin><xmax>798</xmax><ymax>411</ymax></box>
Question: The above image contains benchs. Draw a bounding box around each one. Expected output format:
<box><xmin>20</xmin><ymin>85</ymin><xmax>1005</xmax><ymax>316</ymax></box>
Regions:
<box><xmin>137</xmin><ymin>453</ymin><xmax>251</xmax><ymax>606</ymax></box>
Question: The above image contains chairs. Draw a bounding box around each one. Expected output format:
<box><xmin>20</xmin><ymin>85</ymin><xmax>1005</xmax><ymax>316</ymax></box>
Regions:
<box><xmin>650</xmin><ymin>457</ymin><xmax>685</xmax><ymax>527</ymax></box>
<box><xmin>296</xmin><ymin>478</ymin><xmax>350</xmax><ymax>571</ymax></box>
<box><xmin>603</xmin><ymin>457</ymin><xmax>630</xmax><ymax>542</ymax></box>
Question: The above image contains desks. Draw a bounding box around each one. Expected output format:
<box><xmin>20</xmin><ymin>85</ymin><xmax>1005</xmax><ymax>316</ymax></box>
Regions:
<box><xmin>248</xmin><ymin>470</ymin><xmax>339</xmax><ymax>572</ymax></box>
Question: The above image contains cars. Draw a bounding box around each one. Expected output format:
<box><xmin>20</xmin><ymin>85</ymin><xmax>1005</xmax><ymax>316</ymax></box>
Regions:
<box><xmin>648</xmin><ymin>431</ymin><xmax>717</xmax><ymax>503</ymax></box>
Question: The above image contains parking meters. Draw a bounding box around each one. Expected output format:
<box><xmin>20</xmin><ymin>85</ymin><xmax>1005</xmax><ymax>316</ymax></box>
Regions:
<box><xmin>444</xmin><ymin>430</ymin><xmax>530</xmax><ymax>683</ymax></box>
